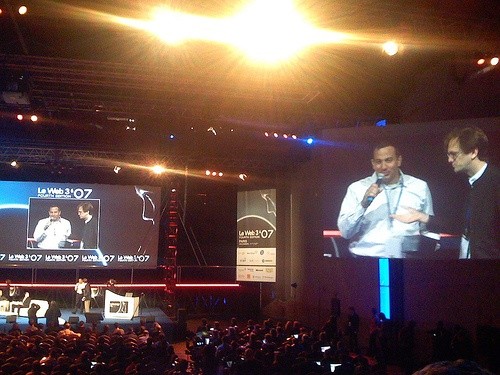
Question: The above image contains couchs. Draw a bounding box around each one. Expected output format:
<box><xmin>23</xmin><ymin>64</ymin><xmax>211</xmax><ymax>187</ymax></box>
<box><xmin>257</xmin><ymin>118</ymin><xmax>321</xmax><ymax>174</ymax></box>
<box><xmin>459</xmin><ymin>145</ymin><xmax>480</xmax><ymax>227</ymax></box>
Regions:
<box><xmin>9</xmin><ymin>300</ymin><xmax>49</xmax><ymax>317</ymax></box>
<box><xmin>0</xmin><ymin>300</ymin><xmax>11</xmax><ymax>311</ymax></box>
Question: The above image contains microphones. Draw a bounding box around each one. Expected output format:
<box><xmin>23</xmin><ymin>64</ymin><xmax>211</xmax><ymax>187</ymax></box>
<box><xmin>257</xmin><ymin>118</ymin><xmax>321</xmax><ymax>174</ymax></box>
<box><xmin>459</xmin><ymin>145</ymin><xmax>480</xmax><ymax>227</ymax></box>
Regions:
<box><xmin>49</xmin><ymin>217</ymin><xmax>53</xmax><ymax>223</ymax></box>
<box><xmin>368</xmin><ymin>173</ymin><xmax>384</xmax><ymax>206</ymax></box>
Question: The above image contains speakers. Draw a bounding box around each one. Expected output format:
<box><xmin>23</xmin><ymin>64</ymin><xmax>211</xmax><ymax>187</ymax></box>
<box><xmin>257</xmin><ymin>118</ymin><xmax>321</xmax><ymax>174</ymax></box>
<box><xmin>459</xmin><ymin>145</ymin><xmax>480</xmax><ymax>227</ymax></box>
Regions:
<box><xmin>68</xmin><ymin>317</ymin><xmax>79</xmax><ymax>323</ymax></box>
<box><xmin>140</xmin><ymin>316</ymin><xmax>156</xmax><ymax>323</ymax></box>
<box><xmin>6</xmin><ymin>316</ymin><xmax>17</xmax><ymax>323</ymax></box>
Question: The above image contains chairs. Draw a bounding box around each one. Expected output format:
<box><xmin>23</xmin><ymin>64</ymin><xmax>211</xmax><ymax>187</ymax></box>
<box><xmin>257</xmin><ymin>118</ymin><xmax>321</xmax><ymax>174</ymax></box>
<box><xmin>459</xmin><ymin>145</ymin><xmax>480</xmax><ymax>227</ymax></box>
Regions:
<box><xmin>0</xmin><ymin>327</ymin><xmax>182</xmax><ymax>375</ymax></box>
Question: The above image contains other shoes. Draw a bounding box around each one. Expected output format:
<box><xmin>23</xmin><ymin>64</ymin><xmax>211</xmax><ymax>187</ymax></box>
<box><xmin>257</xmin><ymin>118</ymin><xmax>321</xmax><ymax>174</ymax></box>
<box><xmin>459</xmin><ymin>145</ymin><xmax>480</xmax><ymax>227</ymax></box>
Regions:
<box><xmin>72</xmin><ymin>312</ymin><xmax>77</xmax><ymax>314</ymax></box>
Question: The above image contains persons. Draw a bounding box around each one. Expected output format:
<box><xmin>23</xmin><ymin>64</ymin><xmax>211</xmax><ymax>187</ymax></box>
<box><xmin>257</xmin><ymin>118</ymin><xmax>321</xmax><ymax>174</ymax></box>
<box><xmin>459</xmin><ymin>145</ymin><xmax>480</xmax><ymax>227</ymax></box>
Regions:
<box><xmin>68</xmin><ymin>203</ymin><xmax>99</xmax><ymax>249</ymax></box>
<box><xmin>337</xmin><ymin>138</ymin><xmax>435</xmax><ymax>260</ymax></box>
<box><xmin>388</xmin><ymin>125</ymin><xmax>500</xmax><ymax>261</ymax></box>
<box><xmin>0</xmin><ymin>277</ymin><xmax>500</xmax><ymax>375</ymax></box>
<box><xmin>33</xmin><ymin>205</ymin><xmax>71</xmax><ymax>249</ymax></box>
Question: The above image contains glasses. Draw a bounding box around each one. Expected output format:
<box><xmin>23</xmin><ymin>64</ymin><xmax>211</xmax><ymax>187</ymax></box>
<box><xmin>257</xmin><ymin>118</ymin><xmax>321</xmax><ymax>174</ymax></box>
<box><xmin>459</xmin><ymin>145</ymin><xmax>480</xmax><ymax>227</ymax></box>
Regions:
<box><xmin>446</xmin><ymin>150</ymin><xmax>466</xmax><ymax>162</ymax></box>
